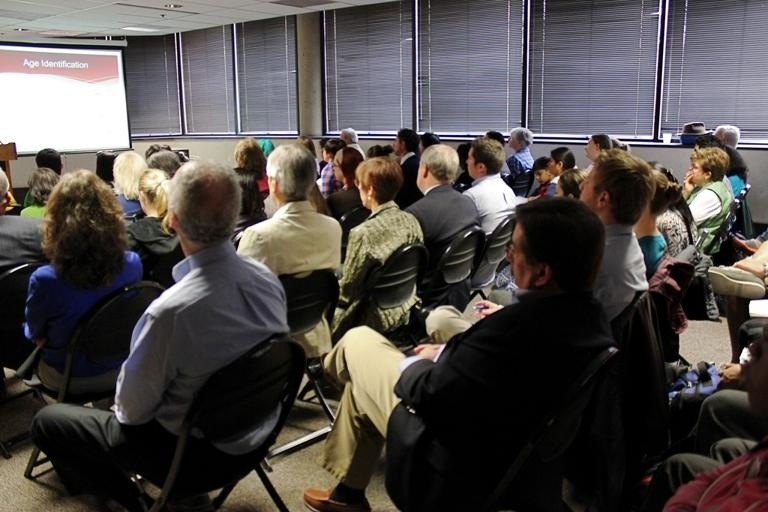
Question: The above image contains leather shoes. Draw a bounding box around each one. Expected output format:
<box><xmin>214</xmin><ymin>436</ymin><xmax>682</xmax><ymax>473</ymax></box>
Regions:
<box><xmin>304</xmin><ymin>487</ymin><xmax>372</xmax><ymax>512</ymax></box>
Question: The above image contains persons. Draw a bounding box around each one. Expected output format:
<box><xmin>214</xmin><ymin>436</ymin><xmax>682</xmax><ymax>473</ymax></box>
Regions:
<box><xmin>459</xmin><ymin>139</ymin><xmax>519</xmax><ymax>292</ymax></box>
<box><xmin>636</xmin><ymin>122</ymin><xmax>768</xmax><ymax>510</ymax></box>
<box><xmin>17</xmin><ymin>168</ymin><xmax>63</xmax><ymax>220</ymax></box>
<box><xmin>584</xmin><ymin>134</ymin><xmax>612</xmax><ymax>174</ymax></box>
<box><xmin>121</xmin><ymin>169</ymin><xmax>187</xmax><ymax>289</ymax></box>
<box><xmin>329</xmin><ymin>156</ymin><xmax>422</xmax><ymax>340</ymax></box>
<box><xmin>107</xmin><ymin>151</ymin><xmax>147</xmax><ymax>222</ymax></box>
<box><xmin>452</xmin><ymin>144</ymin><xmax>475</xmax><ymax>192</ymax></box>
<box><xmin>320</xmin><ymin>138</ymin><xmax>346</xmax><ymax>199</ymax></box>
<box><xmin>232</xmin><ymin>142</ymin><xmax>345</xmax><ymax>359</ymax></box>
<box><xmin>501</xmin><ymin>128</ymin><xmax>533</xmax><ymax>175</ymax></box>
<box><xmin>294</xmin><ymin>136</ymin><xmax>321</xmax><ymax>180</ymax></box>
<box><xmin>234</xmin><ymin>168</ymin><xmax>267</xmax><ymax>238</ymax></box>
<box><xmin>1</xmin><ymin>167</ymin><xmax>52</xmax><ymax>275</ymax></box>
<box><xmin>418</xmin><ymin>133</ymin><xmax>441</xmax><ymax>155</ymax></box>
<box><xmin>94</xmin><ymin>151</ymin><xmax>115</xmax><ymax>185</ymax></box>
<box><xmin>22</xmin><ymin>170</ymin><xmax>142</xmax><ymax>407</ymax></box>
<box><xmin>393</xmin><ymin>128</ymin><xmax>422</xmax><ymax>210</ymax></box>
<box><xmin>302</xmin><ymin>197</ymin><xmax>615</xmax><ymax>511</ymax></box>
<box><xmin>146</xmin><ymin>149</ymin><xmax>182</xmax><ymax>179</ymax></box>
<box><xmin>533</xmin><ymin>148</ymin><xmax>599</xmax><ymax>201</ymax></box>
<box><xmin>33</xmin><ymin>147</ymin><xmax>65</xmax><ymax>178</ymax></box>
<box><xmin>365</xmin><ymin>145</ymin><xmax>393</xmax><ymax>156</ymax></box>
<box><xmin>404</xmin><ymin>145</ymin><xmax>483</xmax><ymax>344</ymax></box>
<box><xmin>235</xmin><ymin>142</ymin><xmax>273</xmax><ymax>200</ymax></box>
<box><xmin>484</xmin><ymin>131</ymin><xmax>512</xmax><ymax>180</ymax></box>
<box><xmin>329</xmin><ymin>147</ymin><xmax>364</xmax><ymax>219</ymax></box>
<box><xmin>423</xmin><ymin>148</ymin><xmax>656</xmax><ymax>344</ymax></box>
<box><xmin>28</xmin><ymin>158</ymin><xmax>290</xmax><ymax>512</ymax></box>
<box><xmin>319</xmin><ymin>137</ymin><xmax>330</xmax><ymax>173</ymax></box>
<box><xmin>609</xmin><ymin>137</ymin><xmax>628</xmax><ymax>152</ymax></box>
<box><xmin>340</xmin><ymin>127</ymin><xmax>366</xmax><ymax>161</ymax></box>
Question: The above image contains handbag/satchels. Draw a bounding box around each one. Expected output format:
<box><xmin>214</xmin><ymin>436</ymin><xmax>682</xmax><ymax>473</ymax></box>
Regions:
<box><xmin>664</xmin><ymin>359</ymin><xmax>721</xmax><ymax>405</ymax></box>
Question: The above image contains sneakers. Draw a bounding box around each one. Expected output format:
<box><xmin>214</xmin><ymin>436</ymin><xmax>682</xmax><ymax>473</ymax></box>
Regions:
<box><xmin>693</xmin><ymin>265</ymin><xmax>766</xmax><ymax>299</ymax></box>
<box><xmin>735</xmin><ymin>238</ymin><xmax>759</xmax><ymax>253</ymax></box>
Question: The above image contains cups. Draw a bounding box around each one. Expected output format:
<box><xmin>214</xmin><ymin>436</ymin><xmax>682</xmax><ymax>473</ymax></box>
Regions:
<box><xmin>662</xmin><ymin>132</ymin><xmax>672</xmax><ymax>143</ymax></box>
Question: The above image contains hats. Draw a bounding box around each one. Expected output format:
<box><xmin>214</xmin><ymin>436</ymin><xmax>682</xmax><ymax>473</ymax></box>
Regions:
<box><xmin>678</xmin><ymin>122</ymin><xmax>709</xmax><ymax>135</ymax></box>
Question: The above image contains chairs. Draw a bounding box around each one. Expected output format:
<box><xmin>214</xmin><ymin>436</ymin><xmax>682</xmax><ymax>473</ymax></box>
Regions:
<box><xmin>332</xmin><ymin>245</ymin><xmax>428</xmax><ymax>358</ymax></box>
<box><xmin>0</xmin><ymin>262</ymin><xmax>45</xmax><ymax>459</ymax></box>
<box><xmin>106</xmin><ymin>334</ymin><xmax>306</xmax><ymax>511</ymax></box>
<box><xmin>25</xmin><ymin>279</ymin><xmax>167</xmax><ymax>479</ymax></box>
<box><xmin>625</xmin><ymin>181</ymin><xmax>762</xmax><ymax>387</ymax></box>
<box><xmin>424</xmin><ymin>225</ymin><xmax>489</xmax><ymax>314</ymax></box>
<box><xmin>266</xmin><ymin>269</ymin><xmax>344</xmax><ymax>456</ymax></box>
<box><xmin>466</xmin><ymin>216</ymin><xmax>516</xmax><ymax>301</ymax></box>
<box><xmin>487</xmin><ymin>346</ymin><xmax>623</xmax><ymax>511</ymax></box>
<box><xmin>341</xmin><ymin>207</ymin><xmax>373</xmax><ymax>259</ymax></box>
<box><xmin>509</xmin><ymin>168</ymin><xmax>535</xmax><ymax>198</ymax></box>
